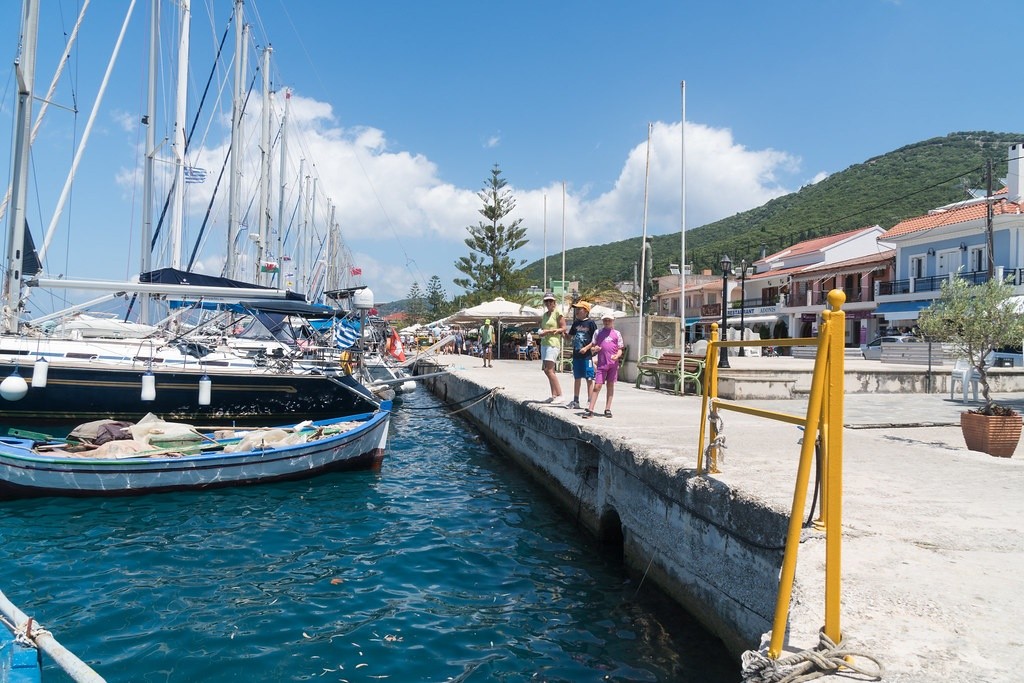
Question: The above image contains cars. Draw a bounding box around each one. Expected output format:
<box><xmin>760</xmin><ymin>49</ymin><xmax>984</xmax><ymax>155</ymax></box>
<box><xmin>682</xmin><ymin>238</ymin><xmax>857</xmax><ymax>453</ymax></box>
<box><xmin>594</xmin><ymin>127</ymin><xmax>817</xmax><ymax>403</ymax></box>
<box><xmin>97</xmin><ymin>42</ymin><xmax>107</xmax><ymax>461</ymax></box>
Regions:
<box><xmin>860</xmin><ymin>336</ymin><xmax>921</xmax><ymax>360</ymax></box>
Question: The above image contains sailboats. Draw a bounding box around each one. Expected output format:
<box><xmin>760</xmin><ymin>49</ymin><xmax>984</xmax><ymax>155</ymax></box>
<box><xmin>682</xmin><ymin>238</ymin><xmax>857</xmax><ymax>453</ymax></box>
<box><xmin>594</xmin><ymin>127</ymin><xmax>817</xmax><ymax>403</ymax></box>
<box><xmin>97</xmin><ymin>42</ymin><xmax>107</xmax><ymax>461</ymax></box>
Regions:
<box><xmin>1</xmin><ymin>0</ymin><xmax>403</xmax><ymax>497</ymax></box>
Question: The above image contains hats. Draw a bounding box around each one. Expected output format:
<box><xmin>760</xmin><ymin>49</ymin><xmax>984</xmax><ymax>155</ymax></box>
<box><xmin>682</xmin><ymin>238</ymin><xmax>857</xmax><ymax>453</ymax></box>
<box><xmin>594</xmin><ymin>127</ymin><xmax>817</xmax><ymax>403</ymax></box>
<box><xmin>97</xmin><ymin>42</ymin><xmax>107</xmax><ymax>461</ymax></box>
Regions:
<box><xmin>485</xmin><ymin>319</ymin><xmax>490</xmax><ymax>324</ymax></box>
<box><xmin>601</xmin><ymin>309</ymin><xmax>614</xmax><ymax>320</ymax></box>
<box><xmin>543</xmin><ymin>292</ymin><xmax>555</xmax><ymax>300</ymax></box>
<box><xmin>572</xmin><ymin>301</ymin><xmax>590</xmax><ymax>312</ymax></box>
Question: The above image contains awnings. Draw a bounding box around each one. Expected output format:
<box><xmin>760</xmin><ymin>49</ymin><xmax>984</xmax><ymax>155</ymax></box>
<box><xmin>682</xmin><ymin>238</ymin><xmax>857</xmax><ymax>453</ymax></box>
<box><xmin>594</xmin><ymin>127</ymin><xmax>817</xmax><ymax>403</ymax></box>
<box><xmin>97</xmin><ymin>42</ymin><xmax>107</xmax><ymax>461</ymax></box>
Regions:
<box><xmin>717</xmin><ymin>315</ymin><xmax>778</xmax><ymax>323</ymax></box>
<box><xmin>872</xmin><ymin>302</ymin><xmax>936</xmax><ymax>315</ymax></box>
<box><xmin>685</xmin><ymin>319</ymin><xmax>698</xmax><ymax>327</ymax></box>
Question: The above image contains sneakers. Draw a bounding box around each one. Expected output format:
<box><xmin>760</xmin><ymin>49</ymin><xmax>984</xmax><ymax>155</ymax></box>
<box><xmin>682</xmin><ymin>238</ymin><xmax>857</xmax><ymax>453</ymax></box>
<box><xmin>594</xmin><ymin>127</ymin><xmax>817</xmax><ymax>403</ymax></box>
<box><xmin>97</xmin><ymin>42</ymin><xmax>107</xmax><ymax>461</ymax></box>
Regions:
<box><xmin>565</xmin><ymin>402</ymin><xmax>579</xmax><ymax>409</ymax></box>
<box><xmin>585</xmin><ymin>402</ymin><xmax>590</xmax><ymax>412</ymax></box>
<box><xmin>604</xmin><ymin>410</ymin><xmax>612</xmax><ymax>417</ymax></box>
<box><xmin>582</xmin><ymin>409</ymin><xmax>593</xmax><ymax>418</ymax></box>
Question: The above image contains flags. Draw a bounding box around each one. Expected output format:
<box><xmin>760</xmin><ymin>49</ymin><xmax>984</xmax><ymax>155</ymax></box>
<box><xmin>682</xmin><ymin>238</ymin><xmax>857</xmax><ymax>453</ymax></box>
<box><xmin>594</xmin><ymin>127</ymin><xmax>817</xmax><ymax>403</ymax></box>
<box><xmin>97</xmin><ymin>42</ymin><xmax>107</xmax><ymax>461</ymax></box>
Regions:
<box><xmin>351</xmin><ymin>268</ymin><xmax>361</xmax><ymax>276</ymax></box>
<box><xmin>338</xmin><ymin>321</ymin><xmax>362</xmax><ymax>349</ymax></box>
<box><xmin>260</xmin><ymin>261</ymin><xmax>280</xmax><ymax>273</ymax></box>
<box><xmin>387</xmin><ymin>327</ymin><xmax>405</xmax><ymax>362</ymax></box>
<box><xmin>184</xmin><ymin>168</ymin><xmax>206</xmax><ymax>183</ymax></box>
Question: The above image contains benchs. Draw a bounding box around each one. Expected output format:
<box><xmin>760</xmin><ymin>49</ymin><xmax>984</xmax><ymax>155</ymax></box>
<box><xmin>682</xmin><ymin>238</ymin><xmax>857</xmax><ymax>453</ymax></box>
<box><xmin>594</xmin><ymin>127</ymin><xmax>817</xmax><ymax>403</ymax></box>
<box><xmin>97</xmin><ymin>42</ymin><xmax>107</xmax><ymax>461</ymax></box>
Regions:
<box><xmin>619</xmin><ymin>345</ymin><xmax>629</xmax><ymax>381</ymax></box>
<box><xmin>542</xmin><ymin>349</ymin><xmax>574</xmax><ymax>372</ymax></box>
<box><xmin>635</xmin><ymin>352</ymin><xmax>706</xmax><ymax>397</ymax></box>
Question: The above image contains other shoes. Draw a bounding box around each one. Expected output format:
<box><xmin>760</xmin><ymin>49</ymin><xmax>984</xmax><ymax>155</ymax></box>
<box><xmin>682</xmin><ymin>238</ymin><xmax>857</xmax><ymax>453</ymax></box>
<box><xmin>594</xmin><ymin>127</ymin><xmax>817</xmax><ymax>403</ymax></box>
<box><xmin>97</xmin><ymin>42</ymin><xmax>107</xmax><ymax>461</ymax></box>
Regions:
<box><xmin>545</xmin><ymin>398</ymin><xmax>553</xmax><ymax>403</ymax></box>
<box><xmin>551</xmin><ymin>396</ymin><xmax>563</xmax><ymax>404</ymax></box>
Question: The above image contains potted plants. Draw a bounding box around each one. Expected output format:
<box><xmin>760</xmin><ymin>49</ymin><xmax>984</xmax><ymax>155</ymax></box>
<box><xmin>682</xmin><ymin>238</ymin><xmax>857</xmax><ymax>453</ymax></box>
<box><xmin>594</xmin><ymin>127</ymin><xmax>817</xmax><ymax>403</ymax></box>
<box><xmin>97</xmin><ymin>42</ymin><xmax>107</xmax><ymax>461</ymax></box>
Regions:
<box><xmin>914</xmin><ymin>263</ymin><xmax>1024</xmax><ymax>458</ymax></box>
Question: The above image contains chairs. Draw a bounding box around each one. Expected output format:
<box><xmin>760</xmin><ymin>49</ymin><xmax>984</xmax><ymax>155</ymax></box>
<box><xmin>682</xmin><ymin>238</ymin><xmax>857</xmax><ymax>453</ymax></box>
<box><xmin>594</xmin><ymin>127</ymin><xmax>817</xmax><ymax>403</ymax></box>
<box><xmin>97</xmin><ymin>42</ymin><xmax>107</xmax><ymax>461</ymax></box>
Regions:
<box><xmin>516</xmin><ymin>346</ymin><xmax>527</xmax><ymax>360</ymax></box>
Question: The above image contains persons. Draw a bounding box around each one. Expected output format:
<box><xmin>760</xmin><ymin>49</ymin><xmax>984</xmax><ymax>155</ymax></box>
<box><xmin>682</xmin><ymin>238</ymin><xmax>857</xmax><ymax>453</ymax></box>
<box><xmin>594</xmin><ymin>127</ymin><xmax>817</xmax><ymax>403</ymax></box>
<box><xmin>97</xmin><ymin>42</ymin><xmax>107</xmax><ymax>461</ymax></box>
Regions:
<box><xmin>401</xmin><ymin>319</ymin><xmax>495</xmax><ymax>367</ymax></box>
<box><xmin>583</xmin><ymin>313</ymin><xmax>624</xmax><ymax>418</ymax></box>
<box><xmin>560</xmin><ymin>300</ymin><xmax>597</xmax><ymax>410</ymax></box>
<box><xmin>526</xmin><ymin>328</ymin><xmax>535</xmax><ymax>361</ymax></box>
<box><xmin>540</xmin><ymin>293</ymin><xmax>566</xmax><ymax>403</ymax></box>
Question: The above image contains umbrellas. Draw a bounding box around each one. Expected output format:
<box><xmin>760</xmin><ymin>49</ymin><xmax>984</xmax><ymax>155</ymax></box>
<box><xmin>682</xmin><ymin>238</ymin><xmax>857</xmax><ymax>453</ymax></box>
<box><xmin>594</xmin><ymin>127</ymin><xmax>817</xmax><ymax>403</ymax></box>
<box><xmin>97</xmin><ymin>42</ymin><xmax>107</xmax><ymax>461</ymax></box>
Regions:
<box><xmin>398</xmin><ymin>296</ymin><xmax>546</xmax><ymax>359</ymax></box>
<box><xmin>545</xmin><ymin>300</ymin><xmax>626</xmax><ymax>318</ymax></box>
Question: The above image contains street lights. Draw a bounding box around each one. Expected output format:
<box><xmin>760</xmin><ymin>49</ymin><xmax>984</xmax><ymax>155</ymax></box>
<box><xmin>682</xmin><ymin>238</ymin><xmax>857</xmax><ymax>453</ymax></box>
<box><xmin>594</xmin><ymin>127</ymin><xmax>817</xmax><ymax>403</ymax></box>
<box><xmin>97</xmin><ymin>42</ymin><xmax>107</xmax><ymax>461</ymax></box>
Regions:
<box><xmin>738</xmin><ymin>262</ymin><xmax>748</xmax><ymax>357</ymax></box>
<box><xmin>718</xmin><ymin>255</ymin><xmax>731</xmax><ymax>368</ymax></box>
